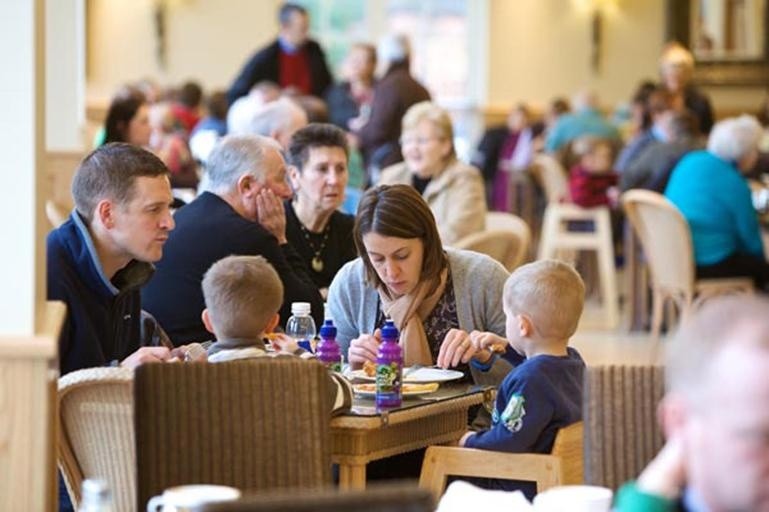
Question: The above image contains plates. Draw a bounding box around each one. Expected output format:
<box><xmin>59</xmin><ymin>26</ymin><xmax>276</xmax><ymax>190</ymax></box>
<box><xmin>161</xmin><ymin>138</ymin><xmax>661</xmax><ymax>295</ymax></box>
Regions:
<box><xmin>347</xmin><ymin>368</ymin><xmax>462</xmax><ymax>382</ymax></box>
<box><xmin>351</xmin><ymin>384</ymin><xmax>431</xmax><ymax>398</ymax></box>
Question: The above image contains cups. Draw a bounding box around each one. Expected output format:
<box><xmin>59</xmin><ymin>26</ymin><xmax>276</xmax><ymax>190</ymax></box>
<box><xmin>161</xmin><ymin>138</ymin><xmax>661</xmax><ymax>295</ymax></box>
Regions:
<box><xmin>143</xmin><ymin>482</ymin><xmax>244</xmax><ymax>511</ymax></box>
<box><xmin>531</xmin><ymin>484</ymin><xmax>615</xmax><ymax>511</ymax></box>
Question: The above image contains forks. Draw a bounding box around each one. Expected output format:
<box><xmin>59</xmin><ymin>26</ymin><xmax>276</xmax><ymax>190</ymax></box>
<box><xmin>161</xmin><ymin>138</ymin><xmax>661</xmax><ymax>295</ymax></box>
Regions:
<box><xmin>406</xmin><ymin>362</ymin><xmax>441</xmax><ymax>377</ymax></box>
<box><xmin>184</xmin><ymin>340</ymin><xmax>213</xmax><ymax>363</ymax></box>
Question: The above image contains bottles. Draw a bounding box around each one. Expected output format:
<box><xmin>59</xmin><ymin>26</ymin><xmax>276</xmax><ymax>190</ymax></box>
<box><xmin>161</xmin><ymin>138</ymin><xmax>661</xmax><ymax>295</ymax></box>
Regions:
<box><xmin>378</xmin><ymin>318</ymin><xmax>403</xmax><ymax>407</ymax></box>
<box><xmin>317</xmin><ymin>319</ymin><xmax>343</xmax><ymax>373</ymax></box>
<box><xmin>77</xmin><ymin>476</ymin><xmax>115</xmax><ymax>511</ymax></box>
<box><xmin>284</xmin><ymin>303</ymin><xmax>317</xmax><ymax>353</ymax></box>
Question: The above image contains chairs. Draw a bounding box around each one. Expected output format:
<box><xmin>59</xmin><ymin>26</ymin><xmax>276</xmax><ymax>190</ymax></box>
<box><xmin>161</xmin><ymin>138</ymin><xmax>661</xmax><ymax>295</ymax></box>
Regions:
<box><xmin>44</xmin><ymin>202</ymin><xmax>76</xmax><ymax>242</ymax></box>
<box><xmin>476</xmin><ymin>206</ymin><xmax>535</xmax><ymax>244</ymax></box>
<box><xmin>132</xmin><ymin>359</ymin><xmax>334</xmax><ymax>510</ymax></box>
<box><xmin>449</xmin><ymin>227</ymin><xmax>521</xmax><ymax>271</ymax></box>
<box><xmin>624</xmin><ymin>194</ymin><xmax>756</xmax><ymax>335</ymax></box>
<box><xmin>538</xmin><ymin>154</ymin><xmax>623</xmax><ymax>325</ymax></box>
<box><xmin>203</xmin><ymin>483</ymin><xmax>436</xmax><ymax>512</ymax></box>
<box><xmin>50</xmin><ymin>369</ymin><xmax>146</xmax><ymax>510</ymax></box>
<box><xmin>583</xmin><ymin>366</ymin><xmax>666</xmax><ymax>489</ymax></box>
<box><xmin>411</xmin><ymin>420</ymin><xmax>583</xmax><ymax>512</ymax></box>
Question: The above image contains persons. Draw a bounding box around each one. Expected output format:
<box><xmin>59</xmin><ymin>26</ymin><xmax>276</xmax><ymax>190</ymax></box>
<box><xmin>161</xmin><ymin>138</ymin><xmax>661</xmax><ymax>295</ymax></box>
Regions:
<box><xmin>613</xmin><ymin>296</ymin><xmax>767</xmax><ymax>512</ymax></box>
<box><xmin>199</xmin><ymin>256</ymin><xmax>358</xmax><ymax>415</ymax></box>
<box><xmin>95</xmin><ymin>3</ymin><xmax>485</xmax><ymax>346</ymax></box>
<box><xmin>458</xmin><ymin>259</ymin><xmax>590</xmax><ymax>500</ymax></box>
<box><xmin>474</xmin><ymin>45</ymin><xmax>768</xmax><ymax>284</ymax></box>
<box><xmin>47</xmin><ymin>141</ymin><xmax>205</xmax><ymax>512</ymax></box>
<box><xmin>322</xmin><ymin>184</ymin><xmax>531</xmax><ymax>438</ymax></box>
